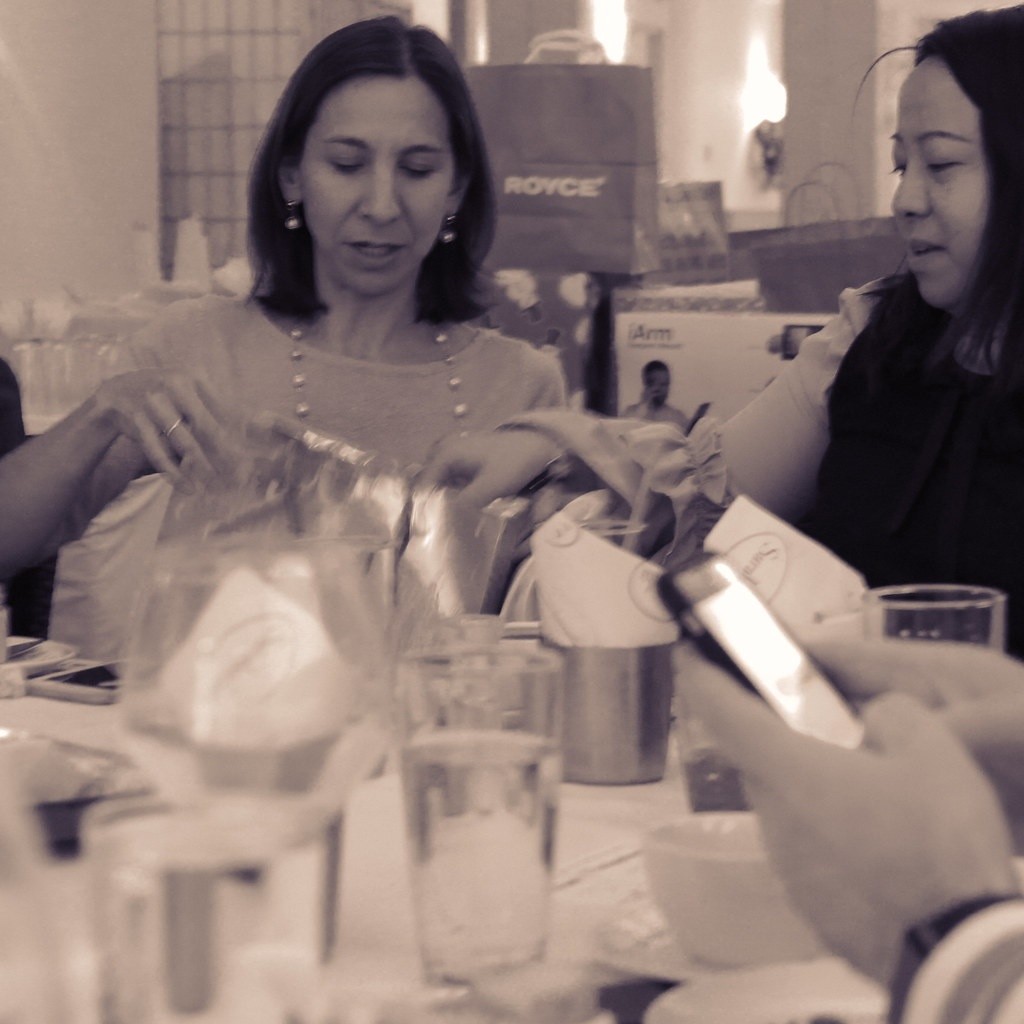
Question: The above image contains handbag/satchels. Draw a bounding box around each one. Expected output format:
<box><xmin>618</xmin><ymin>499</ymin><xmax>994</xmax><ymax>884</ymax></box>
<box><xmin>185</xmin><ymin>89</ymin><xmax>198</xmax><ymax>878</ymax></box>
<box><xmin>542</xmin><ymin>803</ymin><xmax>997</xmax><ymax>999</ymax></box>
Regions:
<box><xmin>754</xmin><ymin>164</ymin><xmax>910</xmax><ymax>312</ymax></box>
<box><xmin>632</xmin><ymin>180</ymin><xmax>732</xmax><ymax>287</ymax></box>
<box><xmin>461</xmin><ymin>30</ymin><xmax>656</xmax><ymax>272</ymax></box>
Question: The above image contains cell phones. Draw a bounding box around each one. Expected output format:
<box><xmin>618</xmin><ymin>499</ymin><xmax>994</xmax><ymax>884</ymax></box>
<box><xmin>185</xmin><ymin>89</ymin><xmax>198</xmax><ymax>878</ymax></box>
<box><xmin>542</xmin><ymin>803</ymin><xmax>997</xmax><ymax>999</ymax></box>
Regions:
<box><xmin>28</xmin><ymin>658</ymin><xmax>128</xmax><ymax>706</ymax></box>
<box><xmin>651</xmin><ymin>552</ymin><xmax>878</xmax><ymax>753</ymax></box>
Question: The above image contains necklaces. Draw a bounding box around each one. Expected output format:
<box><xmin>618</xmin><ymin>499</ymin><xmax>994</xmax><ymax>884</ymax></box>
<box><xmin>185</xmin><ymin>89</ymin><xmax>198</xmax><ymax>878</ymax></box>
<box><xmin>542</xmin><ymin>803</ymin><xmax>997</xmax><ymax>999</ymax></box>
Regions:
<box><xmin>289</xmin><ymin>312</ymin><xmax>474</xmax><ymax>442</ymax></box>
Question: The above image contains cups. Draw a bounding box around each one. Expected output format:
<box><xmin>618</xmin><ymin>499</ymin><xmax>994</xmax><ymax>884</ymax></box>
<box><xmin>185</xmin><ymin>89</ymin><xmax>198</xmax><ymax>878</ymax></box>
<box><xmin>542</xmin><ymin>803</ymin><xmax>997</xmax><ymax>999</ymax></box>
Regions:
<box><xmin>536</xmin><ymin>629</ymin><xmax>678</xmax><ymax>790</ymax></box>
<box><xmin>403</xmin><ymin>646</ymin><xmax>558</xmax><ymax>970</ymax></box>
<box><xmin>860</xmin><ymin>586</ymin><xmax>1007</xmax><ymax>650</ymax></box>
<box><xmin>311</xmin><ymin>532</ymin><xmax>413</xmax><ymax>768</ymax></box>
<box><xmin>82</xmin><ymin>797</ymin><xmax>344</xmax><ymax>1024</ymax></box>
<box><xmin>119</xmin><ymin>540</ymin><xmax>346</xmax><ymax>803</ymax></box>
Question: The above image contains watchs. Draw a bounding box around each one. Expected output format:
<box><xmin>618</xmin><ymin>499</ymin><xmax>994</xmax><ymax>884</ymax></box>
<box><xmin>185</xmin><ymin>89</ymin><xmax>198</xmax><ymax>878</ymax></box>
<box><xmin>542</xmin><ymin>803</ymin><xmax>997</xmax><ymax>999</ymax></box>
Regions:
<box><xmin>884</xmin><ymin>894</ymin><xmax>1020</xmax><ymax>1024</ymax></box>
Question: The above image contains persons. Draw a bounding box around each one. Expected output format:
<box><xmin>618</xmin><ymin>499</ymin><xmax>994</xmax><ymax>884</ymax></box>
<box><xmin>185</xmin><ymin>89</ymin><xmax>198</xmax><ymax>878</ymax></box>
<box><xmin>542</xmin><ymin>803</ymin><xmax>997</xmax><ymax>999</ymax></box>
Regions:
<box><xmin>431</xmin><ymin>4</ymin><xmax>1024</xmax><ymax>665</ymax></box>
<box><xmin>624</xmin><ymin>360</ymin><xmax>691</xmax><ymax>434</ymax></box>
<box><xmin>0</xmin><ymin>13</ymin><xmax>570</xmax><ymax>669</ymax></box>
<box><xmin>669</xmin><ymin>631</ymin><xmax>1024</xmax><ymax>1024</ymax></box>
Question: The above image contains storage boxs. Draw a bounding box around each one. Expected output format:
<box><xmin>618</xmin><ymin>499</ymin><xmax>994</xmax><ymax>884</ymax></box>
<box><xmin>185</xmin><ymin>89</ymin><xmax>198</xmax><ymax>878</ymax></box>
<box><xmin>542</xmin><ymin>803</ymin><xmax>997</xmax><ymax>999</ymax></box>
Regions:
<box><xmin>612</xmin><ymin>308</ymin><xmax>840</xmax><ymax>435</ymax></box>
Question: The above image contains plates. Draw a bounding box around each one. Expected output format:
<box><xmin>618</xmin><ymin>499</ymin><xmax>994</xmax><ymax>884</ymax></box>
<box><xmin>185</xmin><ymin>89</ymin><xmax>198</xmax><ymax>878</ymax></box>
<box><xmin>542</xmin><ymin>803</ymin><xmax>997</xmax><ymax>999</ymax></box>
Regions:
<box><xmin>644</xmin><ymin>963</ymin><xmax>890</xmax><ymax>1024</ymax></box>
<box><xmin>0</xmin><ymin>636</ymin><xmax>76</xmax><ymax>677</ymax></box>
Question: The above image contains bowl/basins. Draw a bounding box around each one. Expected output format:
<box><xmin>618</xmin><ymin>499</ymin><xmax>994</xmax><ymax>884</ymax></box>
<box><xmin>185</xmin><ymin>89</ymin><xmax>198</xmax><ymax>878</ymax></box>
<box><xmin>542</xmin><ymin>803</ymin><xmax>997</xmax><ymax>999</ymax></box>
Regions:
<box><xmin>639</xmin><ymin>811</ymin><xmax>822</xmax><ymax>964</ymax></box>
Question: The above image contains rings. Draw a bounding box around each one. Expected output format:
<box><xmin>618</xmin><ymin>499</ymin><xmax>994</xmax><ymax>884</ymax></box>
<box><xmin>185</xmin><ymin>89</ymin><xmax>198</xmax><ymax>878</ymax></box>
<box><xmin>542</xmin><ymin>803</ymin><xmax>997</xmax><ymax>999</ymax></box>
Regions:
<box><xmin>158</xmin><ymin>414</ymin><xmax>184</xmax><ymax>438</ymax></box>
